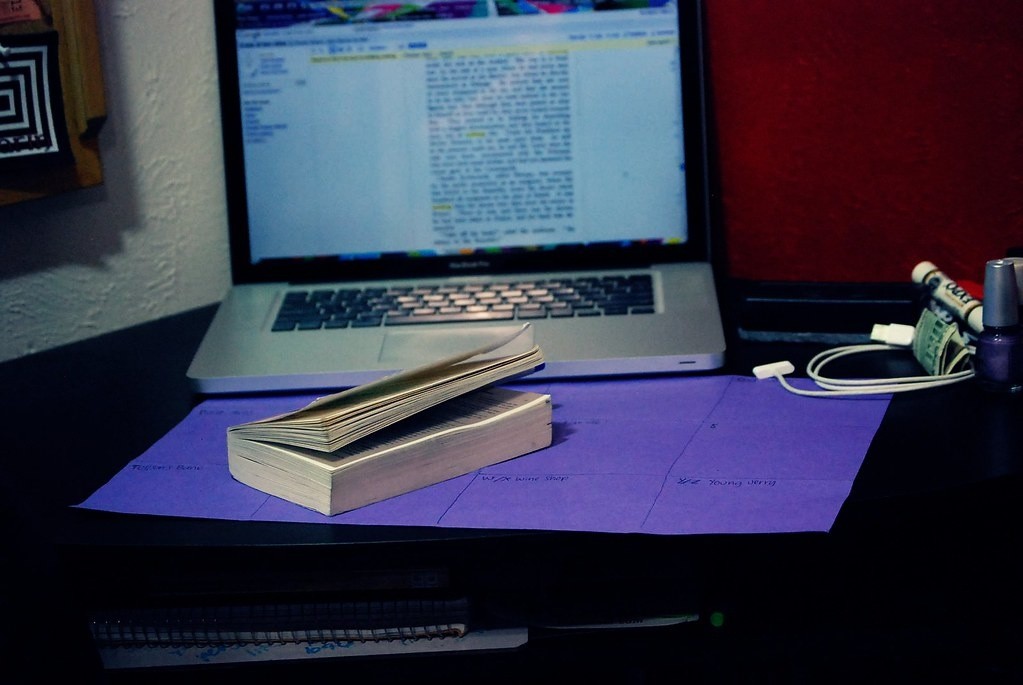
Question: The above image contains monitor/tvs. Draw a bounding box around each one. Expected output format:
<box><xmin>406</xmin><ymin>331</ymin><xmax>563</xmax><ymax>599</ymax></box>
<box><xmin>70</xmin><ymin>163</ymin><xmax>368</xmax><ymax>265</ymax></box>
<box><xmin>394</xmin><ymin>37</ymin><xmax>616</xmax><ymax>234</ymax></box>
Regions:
<box><xmin>698</xmin><ymin>1</ymin><xmax>1023</xmax><ymax>381</ymax></box>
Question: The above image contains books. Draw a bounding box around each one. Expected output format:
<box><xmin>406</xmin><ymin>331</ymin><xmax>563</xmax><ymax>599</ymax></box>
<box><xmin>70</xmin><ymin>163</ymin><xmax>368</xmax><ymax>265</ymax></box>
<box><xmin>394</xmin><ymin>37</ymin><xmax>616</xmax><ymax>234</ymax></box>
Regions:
<box><xmin>227</xmin><ymin>322</ymin><xmax>553</xmax><ymax>517</ymax></box>
<box><xmin>86</xmin><ymin>569</ymin><xmax>473</xmax><ymax>650</ymax></box>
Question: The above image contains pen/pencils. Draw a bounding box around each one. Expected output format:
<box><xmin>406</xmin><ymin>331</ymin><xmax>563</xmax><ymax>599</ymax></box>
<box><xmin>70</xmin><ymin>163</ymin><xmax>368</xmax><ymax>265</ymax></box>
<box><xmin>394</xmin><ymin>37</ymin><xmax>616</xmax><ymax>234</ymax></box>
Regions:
<box><xmin>457</xmin><ymin>361</ymin><xmax>546</xmax><ymax>397</ymax></box>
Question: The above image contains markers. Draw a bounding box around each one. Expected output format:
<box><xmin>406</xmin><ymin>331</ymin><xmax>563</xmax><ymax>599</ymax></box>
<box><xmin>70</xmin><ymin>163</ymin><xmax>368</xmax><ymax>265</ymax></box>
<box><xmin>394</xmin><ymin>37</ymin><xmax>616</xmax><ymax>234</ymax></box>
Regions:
<box><xmin>911</xmin><ymin>261</ymin><xmax>986</xmax><ymax>335</ymax></box>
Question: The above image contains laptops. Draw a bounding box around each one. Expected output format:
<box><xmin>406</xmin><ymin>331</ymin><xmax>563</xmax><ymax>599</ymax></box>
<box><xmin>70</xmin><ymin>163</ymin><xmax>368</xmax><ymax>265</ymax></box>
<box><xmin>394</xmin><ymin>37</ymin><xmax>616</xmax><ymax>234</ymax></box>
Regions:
<box><xmin>186</xmin><ymin>1</ymin><xmax>727</xmax><ymax>394</ymax></box>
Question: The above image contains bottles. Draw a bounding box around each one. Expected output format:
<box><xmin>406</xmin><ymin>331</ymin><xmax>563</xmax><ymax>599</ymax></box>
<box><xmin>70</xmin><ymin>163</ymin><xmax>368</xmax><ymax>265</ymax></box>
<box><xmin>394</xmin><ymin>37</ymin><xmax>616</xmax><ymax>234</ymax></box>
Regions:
<box><xmin>1004</xmin><ymin>257</ymin><xmax>1023</xmax><ymax>331</ymax></box>
<box><xmin>977</xmin><ymin>259</ymin><xmax>1023</xmax><ymax>394</ymax></box>
